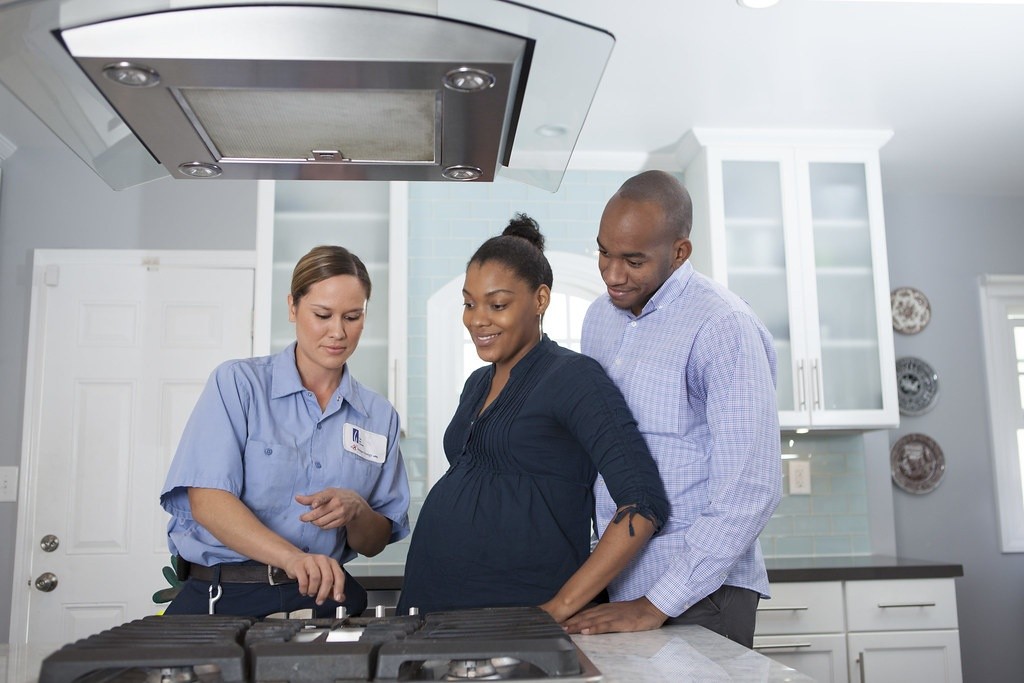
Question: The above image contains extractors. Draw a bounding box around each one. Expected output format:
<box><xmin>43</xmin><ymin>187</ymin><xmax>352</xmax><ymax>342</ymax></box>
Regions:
<box><xmin>2</xmin><ymin>1</ymin><xmax>615</xmax><ymax>198</ymax></box>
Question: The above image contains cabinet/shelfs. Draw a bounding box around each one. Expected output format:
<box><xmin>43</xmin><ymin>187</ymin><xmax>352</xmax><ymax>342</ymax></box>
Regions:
<box><xmin>752</xmin><ymin>581</ymin><xmax>964</xmax><ymax>683</ymax></box>
<box><xmin>671</xmin><ymin>129</ymin><xmax>901</xmax><ymax>430</ymax></box>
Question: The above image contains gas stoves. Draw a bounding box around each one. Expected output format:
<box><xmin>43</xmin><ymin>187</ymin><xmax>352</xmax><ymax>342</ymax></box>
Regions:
<box><xmin>41</xmin><ymin>608</ymin><xmax>604</xmax><ymax>683</ymax></box>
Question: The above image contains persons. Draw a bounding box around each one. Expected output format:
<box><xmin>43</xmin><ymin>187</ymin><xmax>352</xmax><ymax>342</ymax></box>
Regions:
<box><xmin>558</xmin><ymin>170</ymin><xmax>783</xmax><ymax>651</ymax></box>
<box><xmin>160</xmin><ymin>245</ymin><xmax>411</xmax><ymax>617</ymax></box>
<box><xmin>393</xmin><ymin>212</ymin><xmax>671</xmax><ymax>623</ymax></box>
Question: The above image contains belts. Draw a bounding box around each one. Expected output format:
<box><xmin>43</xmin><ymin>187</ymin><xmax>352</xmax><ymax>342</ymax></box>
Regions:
<box><xmin>190</xmin><ymin>563</ymin><xmax>298</xmax><ymax>585</ymax></box>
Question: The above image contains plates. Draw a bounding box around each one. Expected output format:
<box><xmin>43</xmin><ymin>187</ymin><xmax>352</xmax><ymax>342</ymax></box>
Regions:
<box><xmin>891</xmin><ymin>287</ymin><xmax>931</xmax><ymax>335</ymax></box>
<box><xmin>891</xmin><ymin>433</ymin><xmax>945</xmax><ymax>495</ymax></box>
<box><xmin>896</xmin><ymin>357</ymin><xmax>940</xmax><ymax>418</ymax></box>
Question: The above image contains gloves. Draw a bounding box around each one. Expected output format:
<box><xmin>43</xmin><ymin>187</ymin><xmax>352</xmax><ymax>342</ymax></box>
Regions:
<box><xmin>153</xmin><ymin>555</ymin><xmax>186</xmax><ymax>604</ymax></box>
